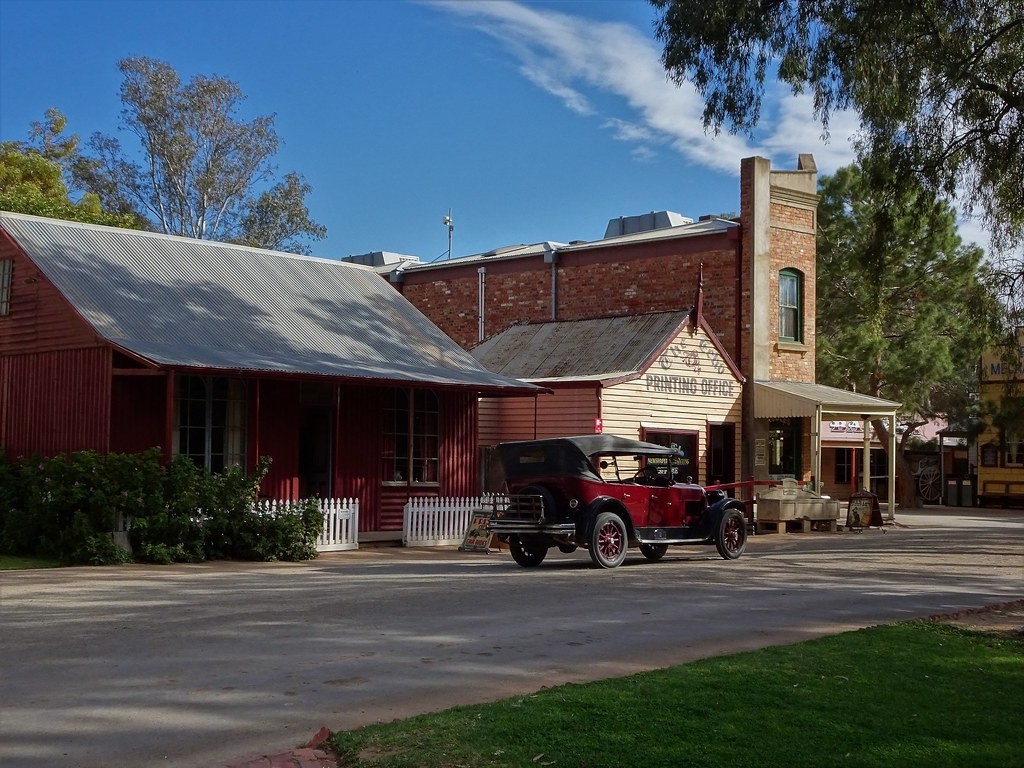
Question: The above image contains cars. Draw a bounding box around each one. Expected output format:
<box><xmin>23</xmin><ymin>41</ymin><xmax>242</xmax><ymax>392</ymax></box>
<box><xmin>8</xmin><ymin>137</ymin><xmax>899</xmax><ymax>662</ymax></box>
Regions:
<box><xmin>486</xmin><ymin>433</ymin><xmax>747</xmax><ymax>568</ymax></box>
